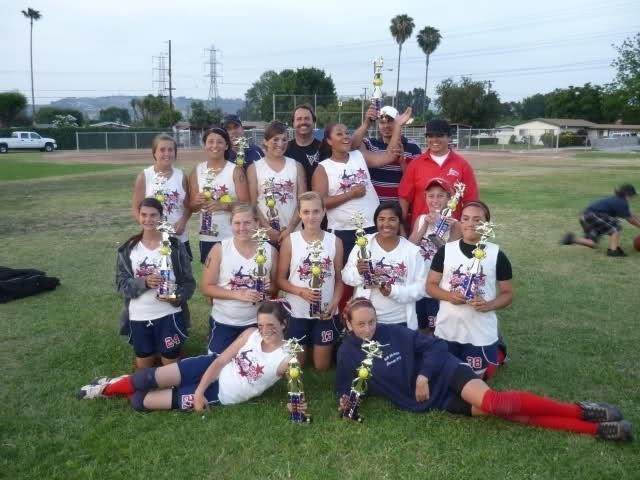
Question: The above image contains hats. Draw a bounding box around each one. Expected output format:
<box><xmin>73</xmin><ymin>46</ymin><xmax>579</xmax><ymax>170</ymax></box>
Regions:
<box><xmin>376</xmin><ymin>105</ymin><xmax>399</xmax><ymax>120</ymax></box>
<box><xmin>218</xmin><ymin>114</ymin><xmax>242</xmax><ymax>130</ymax></box>
<box><xmin>424</xmin><ymin>178</ymin><xmax>451</xmax><ymax>192</ymax></box>
<box><xmin>424</xmin><ymin>119</ymin><xmax>452</xmax><ymax>137</ymax></box>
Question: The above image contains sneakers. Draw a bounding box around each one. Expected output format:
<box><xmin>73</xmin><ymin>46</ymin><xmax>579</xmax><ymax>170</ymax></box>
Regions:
<box><xmin>574</xmin><ymin>400</ymin><xmax>625</xmax><ymax>422</ymax></box>
<box><xmin>559</xmin><ymin>231</ymin><xmax>574</xmax><ymax>246</ymax></box>
<box><xmin>591</xmin><ymin>420</ymin><xmax>633</xmax><ymax>442</ymax></box>
<box><xmin>605</xmin><ymin>246</ymin><xmax>628</xmax><ymax>258</ymax></box>
<box><xmin>75</xmin><ymin>375</ymin><xmax>112</xmax><ymax>401</ymax></box>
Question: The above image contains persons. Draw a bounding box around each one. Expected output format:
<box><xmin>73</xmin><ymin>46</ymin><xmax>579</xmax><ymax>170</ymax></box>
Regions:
<box><xmin>76</xmin><ymin>298</ymin><xmax>308</xmax><ymax>415</ymax></box>
<box><xmin>562</xmin><ymin>184</ymin><xmax>640</xmax><ymax>258</ymax></box>
<box><xmin>334</xmin><ymin>296</ymin><xmax>634</xmax><ymax>444</ymax></box>
<box><xmin>191</xmin><ymin>104</ymin><xmax>514</xmax><ymax>379</ymax></box>
<box><xmin>116</xmin><ymin>199</ymin><xmax>196</xmax><ymax>371</ymax></box>
<box><xmin>133</xmin><ymin>135</ymin><xmax>193</xmax><ymax>260</ymax></box>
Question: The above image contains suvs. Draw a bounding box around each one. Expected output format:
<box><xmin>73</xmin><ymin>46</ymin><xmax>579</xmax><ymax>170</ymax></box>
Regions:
<box><xmin>601</xmin><ymin>132</ymin><xmax>631</xmax><ymax>142</ymax></box>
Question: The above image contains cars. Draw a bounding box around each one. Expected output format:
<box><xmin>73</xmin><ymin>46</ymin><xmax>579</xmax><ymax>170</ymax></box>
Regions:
<box><xmin>630</xmin><ymin>132</ymin><xmax>637</xmax><ymax>136</ymax></box>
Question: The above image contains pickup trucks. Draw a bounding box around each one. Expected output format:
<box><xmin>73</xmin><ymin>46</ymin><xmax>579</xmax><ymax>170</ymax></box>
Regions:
<box><xmin>0</xmin><ymin>131</ymin><xmax>58</xmax><ymax>153</ymax></box>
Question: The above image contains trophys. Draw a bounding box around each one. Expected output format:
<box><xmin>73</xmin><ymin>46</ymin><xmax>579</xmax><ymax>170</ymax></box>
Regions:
<box><xmin>155</xmin><ymin>220</ymin><xmax>176</xmax><ymax>299</ymax></box>
<box><xmin>337</xmin><ymin>337</ymin><xmax>391</xmax><ymax>423</ymax></box>
<box><xmin>152</xmin><ymin>172</ymin><xmax>167</xmax><ymax>222</ymax></box>
<box><xmin>278</xmin><ymin>331</ymin><xmax>313</xmax><ymax>425</ymax></box>
<box><xmin>369</xmin><ymin>56</ymin><xmax>386</xmax><ymax>121</ymax></box>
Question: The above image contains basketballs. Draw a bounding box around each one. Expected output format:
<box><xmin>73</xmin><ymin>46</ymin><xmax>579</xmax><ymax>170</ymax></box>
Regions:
<box><xmin>634</xmin><ymin>235</ymin><xmax>639</xmax><ymax>252</ymax></box>
